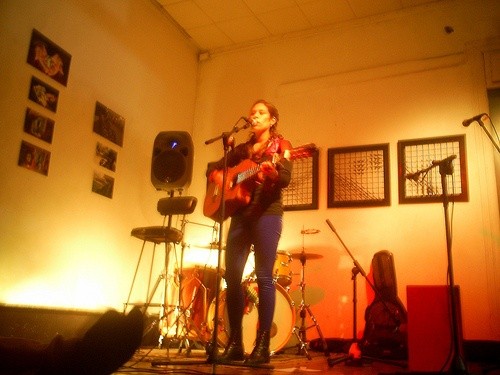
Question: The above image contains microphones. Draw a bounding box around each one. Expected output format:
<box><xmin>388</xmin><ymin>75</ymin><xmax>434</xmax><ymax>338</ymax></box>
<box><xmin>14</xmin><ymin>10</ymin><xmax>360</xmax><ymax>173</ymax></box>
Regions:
<box><xmin>462</xmin><ymin>113</ymin><xmax>485</xmax><ymax>127</ymax></box>
<box><xmin>405</xmin><ymin>173</ymin><xmax>420</xmax><ymax>181</ymax></box>
<box><xmin>242</xmin><ymin>116</ymin><xmax>255</xmax><ymax>126</ymax></box>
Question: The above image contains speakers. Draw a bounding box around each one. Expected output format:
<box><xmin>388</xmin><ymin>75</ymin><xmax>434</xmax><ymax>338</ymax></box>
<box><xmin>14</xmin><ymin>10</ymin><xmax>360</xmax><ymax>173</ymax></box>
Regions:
<box><xmin>150</xmin><ymin>131</ymin><xmax>195</xmax><ymax>192</ymax></box>
<box><xmin>66</xmin><ymin>309</ymin><xmax>144</xmax><ymax>375</ymax></box>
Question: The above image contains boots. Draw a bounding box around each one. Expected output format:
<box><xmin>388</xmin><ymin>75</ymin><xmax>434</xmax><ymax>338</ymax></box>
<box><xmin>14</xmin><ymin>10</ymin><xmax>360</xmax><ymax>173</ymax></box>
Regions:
<box><xmin>245</xmin><ymin>328</ymin><xmax>270</xmax><ymax>367</ymax></box>
<box><xmin>216</xmin><ymin>323</ymin><xmax>244</xmax><ymax>365</ymax></box>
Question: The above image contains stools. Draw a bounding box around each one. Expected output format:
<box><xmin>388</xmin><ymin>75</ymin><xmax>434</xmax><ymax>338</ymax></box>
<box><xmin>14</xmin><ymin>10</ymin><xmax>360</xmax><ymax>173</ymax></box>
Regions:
<box><xmin>124</xmin><ymin>195</ymin><xmax>198</xmax><ymax>359</ymax></box>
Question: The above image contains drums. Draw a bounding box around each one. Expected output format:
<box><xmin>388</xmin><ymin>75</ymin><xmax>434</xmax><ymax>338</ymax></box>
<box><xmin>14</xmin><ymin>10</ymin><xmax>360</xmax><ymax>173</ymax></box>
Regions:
<box><xmin>206</xmin><ymin>277</ymin><xmax>296</xmax><ymax>357</ymax></box>
<box><xmin>160</xmin><ymin>262</ymin><xmax>222</xmax><ymax>343</ymax></box>
<box><xmin>271</xmin><ymin>250</ymin><xmax>292</xmax><ymax>287</ymax></box>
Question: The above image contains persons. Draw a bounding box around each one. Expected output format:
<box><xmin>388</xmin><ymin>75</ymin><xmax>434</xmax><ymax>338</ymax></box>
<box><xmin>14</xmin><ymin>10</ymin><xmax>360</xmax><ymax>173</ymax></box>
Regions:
<box><xmin>206</xmin><ymin>100</ymin><xmax>294</xmax><ymax>365</ymax></box>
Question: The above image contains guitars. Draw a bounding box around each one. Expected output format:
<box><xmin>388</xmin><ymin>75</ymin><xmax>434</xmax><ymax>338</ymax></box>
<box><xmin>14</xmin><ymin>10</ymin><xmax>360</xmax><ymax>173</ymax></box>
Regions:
<box><xmin>203</xmin><ymin>143</ymin><xmax>319</xmax><ymax>223</ymax></box>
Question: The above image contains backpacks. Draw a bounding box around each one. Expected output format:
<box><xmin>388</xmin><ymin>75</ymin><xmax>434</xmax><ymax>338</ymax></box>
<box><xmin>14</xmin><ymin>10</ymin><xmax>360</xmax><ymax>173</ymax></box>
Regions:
<box><xmin>358</xmin><ymin>294</ymin><xmax>407</xmax><ymax>359</ymax></box>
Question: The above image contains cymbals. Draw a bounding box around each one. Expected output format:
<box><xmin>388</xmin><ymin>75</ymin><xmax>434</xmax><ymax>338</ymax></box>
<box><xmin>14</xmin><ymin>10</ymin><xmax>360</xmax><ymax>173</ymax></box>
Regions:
<box><xmin>202</xmin><ymin>245</ymin><xmax>219</xmax><ymax>249</ymax></box>
<box><xmin>291</xmin><ymin>253</ymin><xmax>323</xmax><ymax>259</ymax></box>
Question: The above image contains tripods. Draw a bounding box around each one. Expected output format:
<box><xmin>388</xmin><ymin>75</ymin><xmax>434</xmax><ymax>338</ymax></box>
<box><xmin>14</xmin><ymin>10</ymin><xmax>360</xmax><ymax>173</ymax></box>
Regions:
<box><xmin>295</xmin><ymin>258</ymin><xmax>329</xmax><ymax>357</ymax></box>
<box><xmin>326</xmin><ymin>218</ymin><xmax>407</xmax><ymax>369</ymax></box>
<box><xmin>152</xmin><ymin>123</ymin><xmax>273</xmax><ymax>375</ymax></box>
